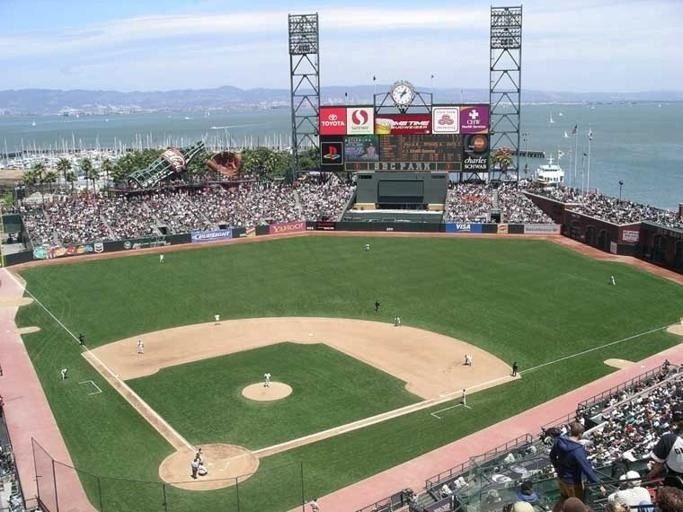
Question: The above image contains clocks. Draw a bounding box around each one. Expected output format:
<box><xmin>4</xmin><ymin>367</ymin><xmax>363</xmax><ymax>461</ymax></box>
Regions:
<box><xmin>389</xmin><ymin>79</ymin><xmax>415</xmax><ymax>108</ymax></box>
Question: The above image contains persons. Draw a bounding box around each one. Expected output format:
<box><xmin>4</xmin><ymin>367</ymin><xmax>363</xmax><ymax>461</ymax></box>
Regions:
<box><xmin>192</xmin><ymin>448</ymin><xmax>210</xmax><ymax>479</ymax></box>
<box><xmin>1</xmin><ymin>170</ymin><xmax>357</xmax><ymax>263</ymax></box>
<box><xmin>607</xmin><ymin>274</ymin><xmax>616</xmax><ymax>287</ymax></box>
<box><xmin>214</xmin><ymin>311</ymin><xmax>222</xmax><ymax>325</ymax></box>
<box><xmin>61</xmin><ymin>368</ymin><xmax>69</xmax><ymax>380</ymax></box>
<box><xmin>79</xmin><ymin>332</ymin><xmax>86</xmax><ymax>346</ymax></box>
<box><xmin>364</xmin><ymin>241</ymin><xmax>371</xmax><ymax>254</ymax></box>
<box><xmin>264</xmin><ymin>371</ymin><xmax>272</xmax><ymax>390</ymax></box>
<box><xmin>136</xmin><ymin>338</ymin><xmax>146</xmax><ymax>360</ymax></box>
<box><xmin>405</xmin><ymin>352</ymin><xmax>683</xmax><ymax>512</ymax></box>
<box><xmin>376</xmin><ymin>300</ymin><xmax>382</xmax><ymax>311</ymax></box>
<box><xmin>363</xmin><ymin>141</ymin><xmax>379</xmax><ymax>161</ymax></box>
<box><xmin>443</xmin><ymin>177</ymin><xmax>683</xmax><ymax>236</ymax></box>
<box><xmin>311</xmin><ymin>498</ymin><xmax>320</xmax><ymax>511</ymax></box>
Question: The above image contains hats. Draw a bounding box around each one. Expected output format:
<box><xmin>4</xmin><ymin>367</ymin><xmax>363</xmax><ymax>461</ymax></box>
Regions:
<box><xmin>626</xmin><ymin>471</ymin><xmax>640</xmax><ymax>485</ymax></box>
<box><xmin>512</xmin><ymin>501</ymin><xmax>533</xmax><ymax>512</ymax></box>
